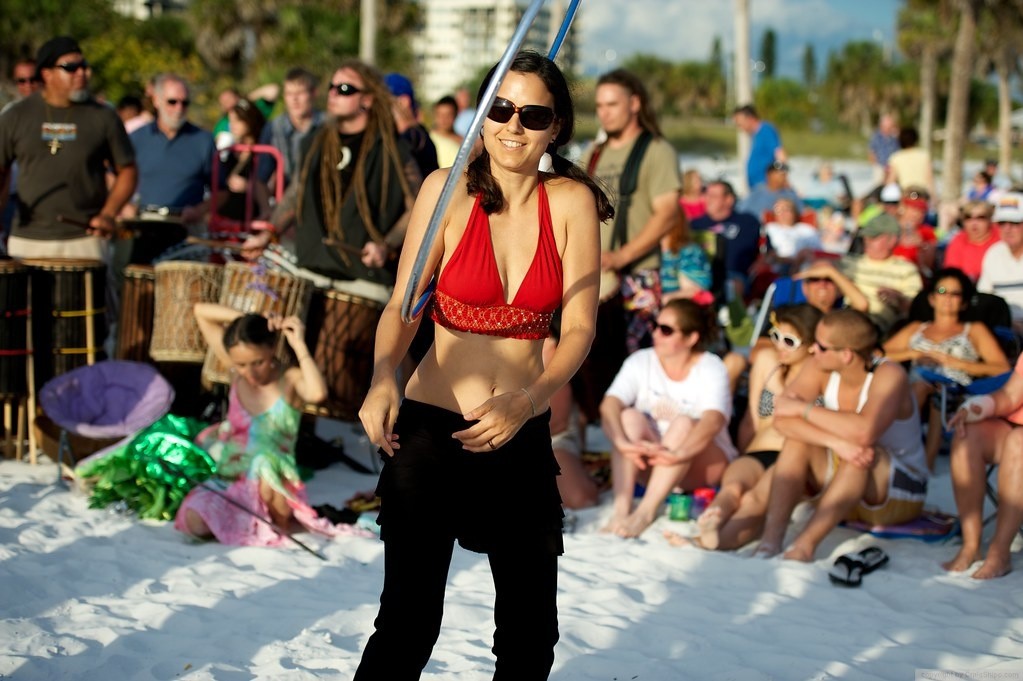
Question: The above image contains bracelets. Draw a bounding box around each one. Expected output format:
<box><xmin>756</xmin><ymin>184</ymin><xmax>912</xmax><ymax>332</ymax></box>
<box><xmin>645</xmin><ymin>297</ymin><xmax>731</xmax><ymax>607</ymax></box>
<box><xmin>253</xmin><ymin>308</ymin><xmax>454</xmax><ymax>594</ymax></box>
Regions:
<box><xmin>804</xmin><ymin>404</ymin><xmax>813</xmax><ymax>421</ymax></box>
<box><xmin>298</xmin><ymin>354</ymin><xmax>311</xmax><ymax>361</ymax></box>
<box><xmin>521</xmin><ymin>388</ymin><xmax>535</xmax><ymax>416</ymax></box>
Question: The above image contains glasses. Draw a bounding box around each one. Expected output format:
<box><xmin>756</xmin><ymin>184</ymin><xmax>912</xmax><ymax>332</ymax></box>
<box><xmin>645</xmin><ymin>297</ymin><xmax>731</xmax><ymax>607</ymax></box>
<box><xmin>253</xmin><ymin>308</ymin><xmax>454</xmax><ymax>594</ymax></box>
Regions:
<box><xmin>997</xmin><ymin>221</ymin><xmax>1021</xmax><ymax>226</ymax></box>
<box><xmin>166</xmin><ymin>97</ymin><xmax>191</xmax><ymax>108</ymax></box>
<box><xmin>933</xmin><ymin>287</ymin><xmax>962</xmax><ymax>297</ymax></box>
<box><xmin>651</xmin><ymin>319</ymin><xmax>675</xmax><ymax>336</ymax></box>
<box><xmin>813</xmin><ymin>337</ymin><xmax>853</xmax><ymax>353</ymax></box>
<box><xmin>13</xmin><ymin>76</ymin><xmax>37</xmax><ymax>85</ymax></box>
<box><xmin>52</xmin><ymin>58</ymin><xmax>87</xmax><ymax>73</ymax></box>
<box><xmin>769</xmin><ymin>325</ymin><xmax>804</xmax><ymax>352</ymax></box>
<box><xmin>485</xmin><ymin>96</ymin><xmax>559</xmax><ymax>131</ymax></box>
<box><xmin>963</xmin><ymin>214</ymin><xmax>987</xmax><ymax>220</ymax></box>
<box><xmin>327</xmin><ymin>80</ymin><xmax>368</xmax><ymax>96</ymax></box>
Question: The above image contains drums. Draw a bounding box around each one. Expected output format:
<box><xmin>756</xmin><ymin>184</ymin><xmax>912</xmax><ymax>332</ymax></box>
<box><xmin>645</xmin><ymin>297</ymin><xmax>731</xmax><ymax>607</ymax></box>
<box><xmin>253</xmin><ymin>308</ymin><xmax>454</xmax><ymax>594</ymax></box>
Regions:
<box><xmin>303</xmin><ymin>289</ymin><xmax>391</xmax><ymax>424</ymax></box>
<box><xmin>202</xmin><ymin>261</ymin><xmax>316</xmax><ymax>387</ymax></box>
<box><xmin>110</xmin><ymin>217</ymin><xmax>189</xmax><ymax>275</ymax></box>
<box><xmin>1</xmin><ymin>257</ymin><xmax>113</xmax><ymax>402</ymax></box>
<box><xmin>148</xmin><ymin>260</ymin><xmax>223</xmax><ymax>363</ymax></box>
<box><xmin>117</xmin><ymin>262</ymin><xmax>174</xmax><ymax>385</ymax></box>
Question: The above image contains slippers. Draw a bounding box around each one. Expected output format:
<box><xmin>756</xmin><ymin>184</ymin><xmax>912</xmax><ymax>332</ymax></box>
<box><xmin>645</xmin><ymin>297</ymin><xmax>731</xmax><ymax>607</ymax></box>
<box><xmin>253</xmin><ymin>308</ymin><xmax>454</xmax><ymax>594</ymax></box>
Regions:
<box><xmin>827</xmin><ymin>554</ymin><xmax>866</xmax><ymax>588</ymax></box>
<box><xmin>857</xmin><ymin>545</ymin><xmax>890</xmax><ymax>575</ymax></box>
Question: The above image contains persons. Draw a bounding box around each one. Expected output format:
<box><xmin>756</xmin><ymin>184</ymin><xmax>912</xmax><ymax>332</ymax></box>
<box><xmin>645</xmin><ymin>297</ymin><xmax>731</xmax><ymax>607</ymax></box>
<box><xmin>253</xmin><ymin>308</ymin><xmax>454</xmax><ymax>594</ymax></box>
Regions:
<box><xmin>175</xmin><ymin>300</ymin><xmax>327</xmax><ymax>539</ymax></box>
<box><xmin>356</xmin><ymin>54</ymin><xmax>613</xmax><ymax>680</ymax></box>
<box><xmin>1</xmin><ymin>36</ymin><xmax>1022</xmax><ymax>578</ymax></box>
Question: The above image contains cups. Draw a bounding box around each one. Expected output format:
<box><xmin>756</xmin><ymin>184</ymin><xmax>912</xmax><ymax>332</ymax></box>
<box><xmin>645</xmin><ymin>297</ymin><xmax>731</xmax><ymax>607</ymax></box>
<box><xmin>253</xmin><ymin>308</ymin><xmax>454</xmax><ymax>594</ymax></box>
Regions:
<box><xmin>668</xmin><ymin>494</ymin><xmax>693</xmax><ymax>520</ymax></box>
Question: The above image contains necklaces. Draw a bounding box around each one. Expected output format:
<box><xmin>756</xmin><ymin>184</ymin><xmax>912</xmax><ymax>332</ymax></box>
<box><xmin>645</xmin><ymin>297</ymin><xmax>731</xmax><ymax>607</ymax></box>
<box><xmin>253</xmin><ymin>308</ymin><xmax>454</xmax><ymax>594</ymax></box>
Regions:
<box><xmin>46</xmin><ymin>106</ymin><xmax>70</xmax><ymax>154</ymax></box>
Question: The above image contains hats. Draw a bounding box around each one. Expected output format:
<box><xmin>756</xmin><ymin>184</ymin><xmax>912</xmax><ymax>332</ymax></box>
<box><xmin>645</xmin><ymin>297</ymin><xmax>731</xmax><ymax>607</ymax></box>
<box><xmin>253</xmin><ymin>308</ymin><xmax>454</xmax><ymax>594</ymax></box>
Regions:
<box><xmin>383</xmin><ymin>71</ymin><xmax>414</xmax><ymax>98</ymax></box>
<box><xmin>899</xmin><ymin>185</ymin><xmax>930</xmax><ymax>211</ymax></box>
<box><xmin>857</xmin><ymin>216</ymin><xmax>902</xmax><ymax>236</ymax></box>
<box><xmin>33</xmin><ymin>34</ymin><xmax>82</xmax><ymax>82</ymax></box>
<box><xmin>989</xmin><ymin>191</ymin><xmax>1023</xmax><ymax>223</ymax></box>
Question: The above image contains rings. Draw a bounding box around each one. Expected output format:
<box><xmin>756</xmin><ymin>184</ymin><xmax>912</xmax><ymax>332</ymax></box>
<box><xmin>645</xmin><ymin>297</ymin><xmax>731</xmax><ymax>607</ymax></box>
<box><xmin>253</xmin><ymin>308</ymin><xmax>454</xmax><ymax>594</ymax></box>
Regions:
<box><xmin>488</xmin><ymin>440</ymin><xmax>496</xmax><ymax>449</ymax></box>
